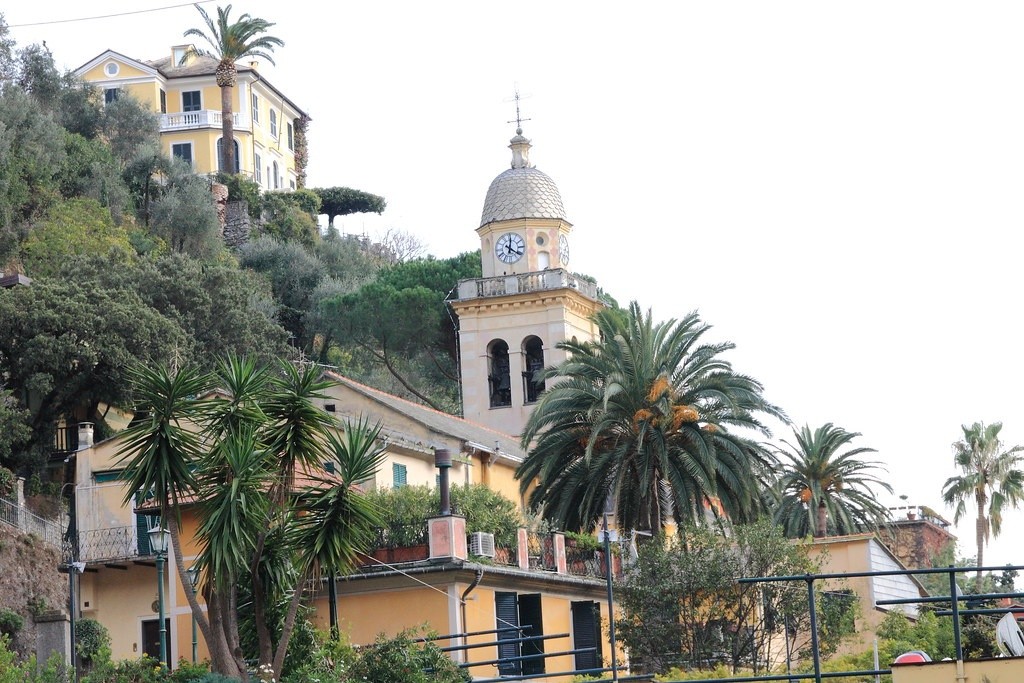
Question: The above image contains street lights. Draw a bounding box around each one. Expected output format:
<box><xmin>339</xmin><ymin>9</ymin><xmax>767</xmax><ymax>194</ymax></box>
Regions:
<box><xmin>186</xmin><ymin>562</ymin><xmax>203</xmax><ymax>665</ymax></box>
<box><xmin>147</xmin><ymin>521</ymin><xmax>171</xmax><ymax>670</ymax></box>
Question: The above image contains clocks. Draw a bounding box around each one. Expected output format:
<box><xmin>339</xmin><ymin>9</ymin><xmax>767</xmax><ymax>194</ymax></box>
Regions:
<box><xmin>494</xmin><ymin>233</ymin><xmax>526</xmax><ymax>264</ymax></box>
<box><xmin>559</xmin><ymin>234</ymin><xmax>570</xmax><ymax>267</ymax></box>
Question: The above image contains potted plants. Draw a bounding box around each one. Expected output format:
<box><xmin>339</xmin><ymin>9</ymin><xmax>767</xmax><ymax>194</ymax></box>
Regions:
<box><xmin>907</xmin><ymin>511</ymin><xmax>916</xmax><ymax>521</ymax></box>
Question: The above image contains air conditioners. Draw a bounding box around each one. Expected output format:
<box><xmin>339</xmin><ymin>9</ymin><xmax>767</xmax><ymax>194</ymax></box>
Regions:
<box><xmin>468</xmin><ymin>531</ymin><xmax>494</xmax><ymax>558</ymax></box>
